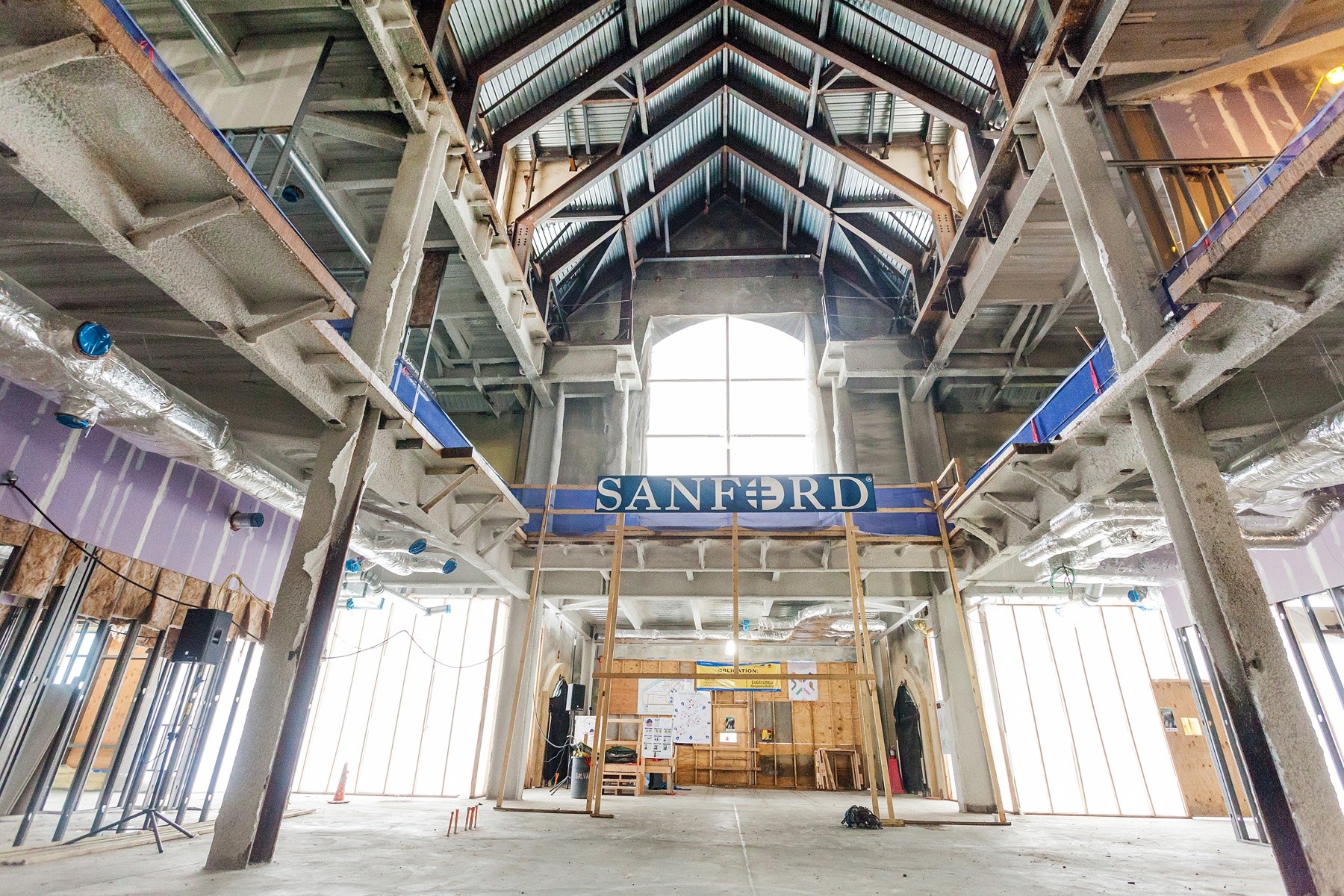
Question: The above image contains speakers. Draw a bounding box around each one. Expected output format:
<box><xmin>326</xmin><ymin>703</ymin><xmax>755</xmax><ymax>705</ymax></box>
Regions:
<box><xmin>172</xmin><ymin>608</ymin><xmax>233</xmax><ymax>664</ymax></box>
<box><xmin>566</xmin><ymin>684</ymin><xmax>586</xmax><ymax>711</ymax></box>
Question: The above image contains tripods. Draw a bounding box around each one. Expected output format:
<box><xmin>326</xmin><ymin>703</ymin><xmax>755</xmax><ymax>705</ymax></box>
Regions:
<box><xmin>64</xmin><ymin>661</ymin><xmax>196</xmax><ymax>854</ymax></box>
<box><xmin>550</xmin><ymin>710</ymin><xmax>575</xmax><ymax>795</ymax></box>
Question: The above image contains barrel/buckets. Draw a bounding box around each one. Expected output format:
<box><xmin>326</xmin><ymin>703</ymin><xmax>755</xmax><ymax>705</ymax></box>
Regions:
<box><xmin>571</xmin><ymin>757</ymin><xmax>598</xmax><ymax>799</ymax></box>
